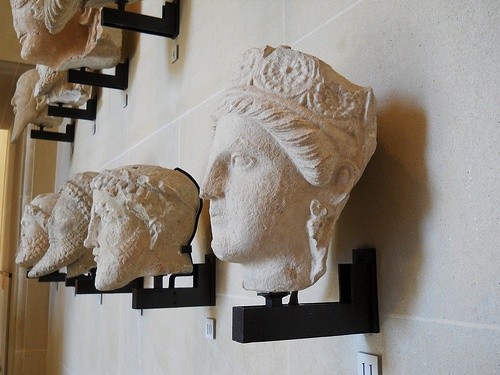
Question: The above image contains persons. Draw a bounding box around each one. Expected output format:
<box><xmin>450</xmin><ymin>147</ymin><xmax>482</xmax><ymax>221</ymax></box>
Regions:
<box><xmin>33</xmin><ymin>62</ymin><xmax>95</xmax><ymax>113</ymax></box>
<box><xmin>83</xmin><ymin>163</ymin><xmax>202</xmax><ymax>293</ymax></box>
<box><xmin>10</xmin><ymin>0</ymin><xmax>122</xmax><ymax>72</ymax></box>
<box><xmin>198</xmin><ymin>44</ymin><xmax>377</xmax><ymax>294</ymax></box>
<box><xmin>10</xmin><ymin>69</ymin><xmax>63</xmax><ymax>145</ymax></box>
<box><xmin>28</xmin><ymin>171</ymin><xmax>103</xmax><ymax>283</ymax></box>
<box><xmin>14</xmin><ymin>190</ymin><xmax>67</xmax><ymax>271</ymax></box>
